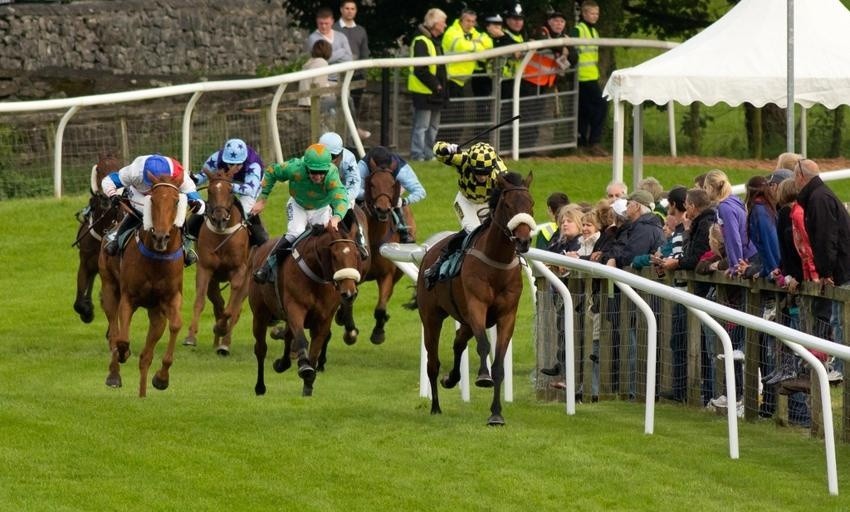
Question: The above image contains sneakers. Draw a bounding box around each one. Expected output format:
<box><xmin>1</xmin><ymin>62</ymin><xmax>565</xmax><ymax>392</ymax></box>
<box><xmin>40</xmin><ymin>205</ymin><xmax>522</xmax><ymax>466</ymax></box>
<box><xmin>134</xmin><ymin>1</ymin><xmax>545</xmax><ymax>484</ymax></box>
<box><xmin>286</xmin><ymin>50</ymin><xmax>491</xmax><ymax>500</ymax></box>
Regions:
<box><xmin>761</xmin><ymin>364</ymin><xmax>810</xmax><ymax>396</ymax></box>
<box><xmin>710</xmin><ymin>396</ymin><xmax>742</xmax><ymax>408</ymax></box>
<box><xmin>716</xmin><ymin>349</ymin><xmax>744</xmax><ymax>360</ymax></box>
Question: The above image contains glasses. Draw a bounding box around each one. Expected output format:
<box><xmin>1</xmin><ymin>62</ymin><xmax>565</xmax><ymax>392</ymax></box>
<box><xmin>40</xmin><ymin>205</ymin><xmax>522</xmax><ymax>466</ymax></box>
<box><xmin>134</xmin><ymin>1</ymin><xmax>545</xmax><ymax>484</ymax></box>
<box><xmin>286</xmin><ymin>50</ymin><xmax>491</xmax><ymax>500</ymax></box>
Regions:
<box><xmin>471</xmin><ymin>166</ymin><xmax>491</xmax><ymax>175</ymax></box>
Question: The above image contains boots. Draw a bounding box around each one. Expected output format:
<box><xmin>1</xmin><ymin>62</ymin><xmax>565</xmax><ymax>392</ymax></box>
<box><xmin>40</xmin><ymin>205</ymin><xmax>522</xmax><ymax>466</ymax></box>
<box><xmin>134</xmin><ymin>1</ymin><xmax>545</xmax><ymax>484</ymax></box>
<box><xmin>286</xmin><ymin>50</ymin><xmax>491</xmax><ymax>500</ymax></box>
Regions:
<box><xmin>392</xmin><ymin>207</ymin><xmax>415</xmax><ymax>243</ymax></box>
<box><xmin>103</xmin><ymin>207</ymin><xmax>143</xmax><ymax>256</ymax></box>
<box><xmin>253</xmin><ymin>234</ymin><xmax>292</xmax><ymax>284</ymax></box>
<box><xmin>424</xmin><ymin>229</ymin><xmax>468</xmax><ymax>291</ymax></box>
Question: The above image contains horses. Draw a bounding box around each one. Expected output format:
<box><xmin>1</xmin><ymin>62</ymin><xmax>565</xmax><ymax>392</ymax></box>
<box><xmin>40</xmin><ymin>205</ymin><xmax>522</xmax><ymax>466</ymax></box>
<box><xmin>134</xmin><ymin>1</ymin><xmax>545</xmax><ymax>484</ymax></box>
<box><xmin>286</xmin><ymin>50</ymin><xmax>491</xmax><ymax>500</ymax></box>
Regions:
<box><xmin>342</xmin><ymin>154</ymin><xmax>417</xmax><ymax>347</ymax></box>
<box><xmin>269</xmin><ymin>198</ymin><xmax>372</xmax><ymax>373</ymax></box>
<box><xmin>72</xmin><ymin>186</ymin><xmax>124</xmax><ymax>324</ymax></box>
<box><xmin>96</xmin><ymin>166</ymin><xmax>186</xmax><ymax>400</ymax></box>
<box><xmin>247</xmin><ymin>220</ymin><xmax>361</xmax><ymax>399</ymax></box>
<box><xmin>400</xmin><ymin>168</ymin><xmax>537</xmax><ymax>428</ymax></box>
<box><xmin>181</xmin><ymin>164</ymin><xmax>252</xmax><ymax>358</ymax></box>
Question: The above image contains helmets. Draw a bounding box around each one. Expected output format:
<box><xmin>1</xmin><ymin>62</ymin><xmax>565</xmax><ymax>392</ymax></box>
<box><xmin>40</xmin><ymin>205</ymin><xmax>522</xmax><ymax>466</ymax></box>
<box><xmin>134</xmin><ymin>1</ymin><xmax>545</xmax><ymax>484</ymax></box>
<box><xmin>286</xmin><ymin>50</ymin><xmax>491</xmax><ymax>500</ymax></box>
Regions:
<box><xmin>467</xmin><ymin>142</ymin><xmax>497</xmax><ymax>171</ymax></box>
<box><xmin>222</xmin><ymin>139</ymin><xmax>248</xmax><ymax>165</ymax></box>
<box><xmin>304</xmin><ymin>132</ymin><xmax>343</xmax><ymax>171</ymax></box>
<box><xmin>143</xmin><ymin>155</ymin><xmax>170</xmax><ymax>187</ymax></box>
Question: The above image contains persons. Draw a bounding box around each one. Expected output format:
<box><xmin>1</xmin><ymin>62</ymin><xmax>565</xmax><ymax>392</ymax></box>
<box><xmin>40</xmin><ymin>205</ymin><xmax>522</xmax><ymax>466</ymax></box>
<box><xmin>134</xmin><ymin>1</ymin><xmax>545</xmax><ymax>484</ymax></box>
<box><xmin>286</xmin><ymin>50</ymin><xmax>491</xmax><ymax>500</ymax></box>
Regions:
<box><xmin>434</xmin><ymin>142</ymin><xmax>508</xmax><ymax>247</ymax></box>
<box><xmin>185</xmin><ymin>138</ymin><xmax>269</xmax><ymax>267</ymax></box>
<box><xmin>407</xmin><ymin>2</ymin><xmax>606</xmax><ymax>161</ymax></box>
<box><xmin>357</xmin><ymin>147</ymin><xmax>425</xmax><ymax>242</ymax></box>
<box><xmin>102</xmin><ymin>155</ymin><xmax>201</xmax><ymax>267</ymax></box>
<box><xmin>319</xmin><ymin>132</ymin><xmax>369</xmax><ymax>260</ymax></box>
<box><xmin>535</xmin><ymin>153</ymin><xmax>848</xmax><ymax>428</ymax></box>
<box><xmin>298</xmin><ymin>2</ymin><xmax>370</xmax><ymax>155</ymax></box>
<box><xmin>252</xmin><ymin>144</ymin><xmax>348</xmax><ymax>283</ymax></box>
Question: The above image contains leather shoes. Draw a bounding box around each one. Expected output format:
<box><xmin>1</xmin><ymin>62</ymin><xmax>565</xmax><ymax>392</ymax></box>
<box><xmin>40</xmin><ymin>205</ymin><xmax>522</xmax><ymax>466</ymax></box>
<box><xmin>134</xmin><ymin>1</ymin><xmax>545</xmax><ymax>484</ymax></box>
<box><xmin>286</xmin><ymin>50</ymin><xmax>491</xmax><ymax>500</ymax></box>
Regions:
<box><xmin>541</xmin><ymin>366</ymin><xmax>560</xmax><ymax>376</ymax></box>
<box><xmin>548</xmin><ymin>381</ymin><xmax>566</xmax><ymax>390</ymax></box>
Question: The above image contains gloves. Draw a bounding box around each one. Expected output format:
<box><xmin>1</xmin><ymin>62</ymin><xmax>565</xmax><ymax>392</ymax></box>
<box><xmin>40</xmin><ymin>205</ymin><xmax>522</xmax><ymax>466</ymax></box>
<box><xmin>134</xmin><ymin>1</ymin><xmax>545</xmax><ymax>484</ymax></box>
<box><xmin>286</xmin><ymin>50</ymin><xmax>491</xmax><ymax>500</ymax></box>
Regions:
<box><xmin>447</xmin><ymin>143</ymin><xmax>462</xmax><ymax>155</ymax></box>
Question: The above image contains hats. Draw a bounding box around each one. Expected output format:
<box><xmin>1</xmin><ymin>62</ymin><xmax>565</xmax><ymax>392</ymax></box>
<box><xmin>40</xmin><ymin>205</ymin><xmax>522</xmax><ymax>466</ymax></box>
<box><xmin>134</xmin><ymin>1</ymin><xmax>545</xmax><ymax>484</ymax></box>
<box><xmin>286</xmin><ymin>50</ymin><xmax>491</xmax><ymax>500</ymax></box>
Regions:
<box><xmin>621</xmin><ymin>189</ymin><xmax>655</xmax><ymax>213</ymax></box>
<box><xmin>764</xmin><ymin>168</ymin><xmax>793</xmax><ymax>184</ymax></box>
<box><xmin>485</xmin><ymin>2</ymin><xmax>528</xmax><ymax>22</ymax></box>
